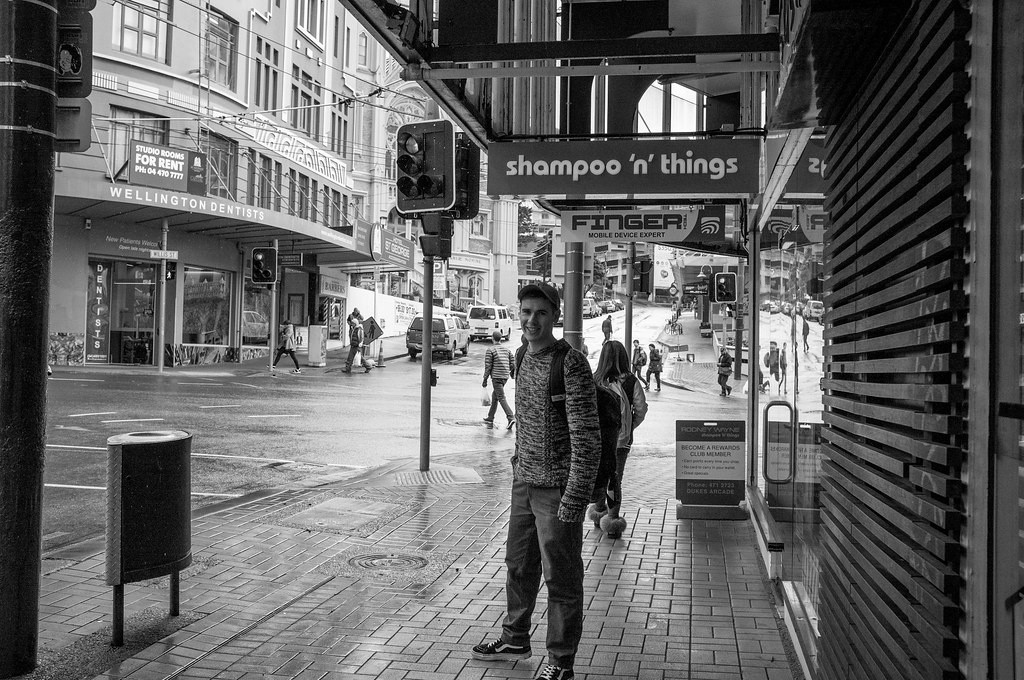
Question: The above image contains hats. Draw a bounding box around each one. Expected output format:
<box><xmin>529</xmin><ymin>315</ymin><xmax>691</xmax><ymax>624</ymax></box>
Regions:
<box><xmin>282</xmin><ymin>320</ymin><xmax>294</xmax><ymax>324</ymax></box>
<box><xmin>517</xmin><ymin>282</ymin><xmax>560</xmax><ymax>310</ymax></box>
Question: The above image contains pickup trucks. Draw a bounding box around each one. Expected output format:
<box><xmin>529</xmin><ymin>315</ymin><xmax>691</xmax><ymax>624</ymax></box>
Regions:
<box><xmin>242</xmin><ymin>311</ymin><xmax>284</xmax><ymax>345</ymax></box>
<box><xmin>406</xmin><ymin>313</ymin><xmax>471</xmax><ymax>360</ymax></box>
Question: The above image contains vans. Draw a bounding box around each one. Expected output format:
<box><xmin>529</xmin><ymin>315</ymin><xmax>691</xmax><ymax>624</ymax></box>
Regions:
<box><xmin>466</xmin><ymin>306</ymin><xmax>514</xmax><ymax>341</ymax></box>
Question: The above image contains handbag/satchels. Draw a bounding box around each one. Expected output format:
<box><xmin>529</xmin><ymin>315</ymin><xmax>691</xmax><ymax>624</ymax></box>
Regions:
<box><xmin>651</xmin><ymin>355</ymin><xmax>662</xmax><ymax>372</ymax></box>
<box><xmin>481</xmin><ymin>386</ymin><xmax>491</xmax><ymax>406</ymax></box>
<box><xmin>639</xmin><ymin>347</ymin><xmax>647</xmax><ymax>365</ymax></box>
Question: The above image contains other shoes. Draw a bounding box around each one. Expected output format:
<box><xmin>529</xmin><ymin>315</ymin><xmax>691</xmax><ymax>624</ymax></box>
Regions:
<box><xmin>365</xmin><ymin>366</ymin><xmax>372</xmax><ymax>373</ymax></box>
<box><xmin>266</xmin><ymin>364</ymin><xmax>275</xmax><ymax>370</ymax></box>
<box><xmin>654</xmin><ymin>388</ymin><xmax>660</xmax><ymax>391</ymax></box>
<box><xmin>293</xmin><ymin>368</ymin><xmax>301</xmax><ymax>373</ymax></box>
<box><xmin>727</xmin><ymin>386</ymin><xmax>732</xmax><ymax>396</ymax></box>
<box><xmin>719</xmin><ymin>394</ymin><xmax>725</xmax><ymax>396</ymax></box>
<box><xmin>483</xmin><ymin>417</ymin><xmax>493</xmax><ymax>423</ymax></box>
<box><xmin>646</xmin><ymin>390</ymin><xmax>649</xmax><ymax>391</ymax></box>
<box><xmin>341</xmin><ymin>369</ymin><xmax>349</xmax><ymax>374</ymax></box>
<box><xmin>506</xmin><ymin>420</ymin><xmax>515</xmax><ymax>429</ymax></box>
<box><xmin>644</xmin><ymin>383</ymin><xmax>650</xmax><ymax>389</ymax></box>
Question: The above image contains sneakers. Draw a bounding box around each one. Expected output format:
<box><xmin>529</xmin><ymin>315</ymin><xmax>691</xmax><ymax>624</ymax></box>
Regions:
<box><xmin>471</xmin><ymin>637</ymin><xmax>533</xmax><ymax>660</ymax></box>
<box><xmin>536</xmin><ymin>664</ymin><xmax>574</xmax><ymax>680</ymax></box>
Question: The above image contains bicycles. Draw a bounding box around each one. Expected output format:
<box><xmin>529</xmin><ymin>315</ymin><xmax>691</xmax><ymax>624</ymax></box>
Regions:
<box><xmin>665</xmin><ymin>318</ymin><xmax>672</xmax><ymax>334</ymax></box>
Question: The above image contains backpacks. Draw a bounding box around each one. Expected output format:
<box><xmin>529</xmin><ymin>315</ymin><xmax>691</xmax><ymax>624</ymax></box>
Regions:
<box><xmin>516</xmin><ymin>340</ymin><xmax>622</xmax><ymax>503</ymax></box>
<box><xmin>599</xmin><ymin>372</ymin><xmax>633</xmax><ymax>448</ymax></box>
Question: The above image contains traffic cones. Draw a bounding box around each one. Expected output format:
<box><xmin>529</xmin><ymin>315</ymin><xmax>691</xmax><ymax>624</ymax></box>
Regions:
<box><xmin>375</xmin><ymin>339</ymin><xmax>386</xmax><ymax>367</ymax></box>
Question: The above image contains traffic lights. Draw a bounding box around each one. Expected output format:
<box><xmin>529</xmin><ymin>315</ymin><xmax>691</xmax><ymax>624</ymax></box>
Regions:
<box><xmin>166</xmin><ymin>261</ymin><xmax>176</xmax><ymax>281</ymax></box>
<box><xmin>715</xmin><ymin>273</ymin><xmax>738</xmax><ymax>303</ymax></box>
<box><xmin>395</xmin><ymin>119</ymin><xmax>456</xmax><ymax>213</ymax></box>
<box><xmin>725</xmin><ymin>304</ymin><xmax>736</xmax><ymax>319</ymax></box>
<box><xmin>251</xmin><ymin>248</ymin><xmax>278</xmax><ymax>284</ymax></box>
<box><xmin>419</xmin><ymin>214</ymin><xmax>453</xmax><ymax>262</ymax></box>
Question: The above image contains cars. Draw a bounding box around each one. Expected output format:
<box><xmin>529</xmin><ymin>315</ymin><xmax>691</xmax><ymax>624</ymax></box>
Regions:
<box><xmin>763</xmin><ymin>300</ymin><xmax>823</xmax><ymax>324</ymax></box>
<box><xmin>553</xmin><ymin>298</ymin><xmax>624</xmax><ymax>327</ymax></box>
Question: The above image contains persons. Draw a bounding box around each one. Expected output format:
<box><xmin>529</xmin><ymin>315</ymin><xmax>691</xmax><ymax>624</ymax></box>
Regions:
<box><xmin>602</xmin><ymin>315</ymin><xmax>613</xmax><ymax>345</ymax></box>
<box><xmin>592</xmin><ymin>340</ymin><xmax>648</xmax><ymax>539</ymax></box>
<box><xmin>341</xmin><ymin>308</ymin><xmax>373</xmax><ymax>374</ymax></box>
<box><xmin>631</xmin><ymin>340</ymin><xmax>650</xmax><ymax>389</ymax></box>
<box><xmin>266</xmin><ymin>320</ymin><xmax>301</xmax><ymax>374</ymax></box>
<box><xmin>717</xmin><ymin>285</ymin><xmax>732</xmax><ymax>301</ymax></box>
<box><xmin>716</xmin><ymin>347</ymin><xmax>732</xmax><ymax>396</ymax></box>
<box><xmin>778</xmin><ymin>342</ymin><xmax>788</xmax><ymax>394</ymax></box>
<box><xmin>770</xmin><ymin>341</ymin><xmax>779</xmax><ymax>381</ymax></box>
<box><xmin>482</xmin><ymin>331</ymin><xmax>516</xmax><ymax>429</ymax></box>
<box><xmin>471</xmin><ymin>280</ymin><xmax>601</xmax><ymax>680</ymax></box>
<box><xmin>803</xmin><ymin>316</ymin><xmax>810</xmax><ymax>352</ymax></box>
<box><xmin>646</xmin><ymin>344</ymin><xmax>662</xmax><ymax>391</ymax></box>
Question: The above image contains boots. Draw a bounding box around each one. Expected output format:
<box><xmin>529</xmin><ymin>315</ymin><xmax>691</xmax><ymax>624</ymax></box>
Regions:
<box><xmin>600</xmin><ymin>513</ymin><xmax>626</xmax><ymax>538</ymax></box>
<box><xmin>587</xmin><ymin>505</ymin><xmax>608</xmax><ymax>528</ymax></box>
